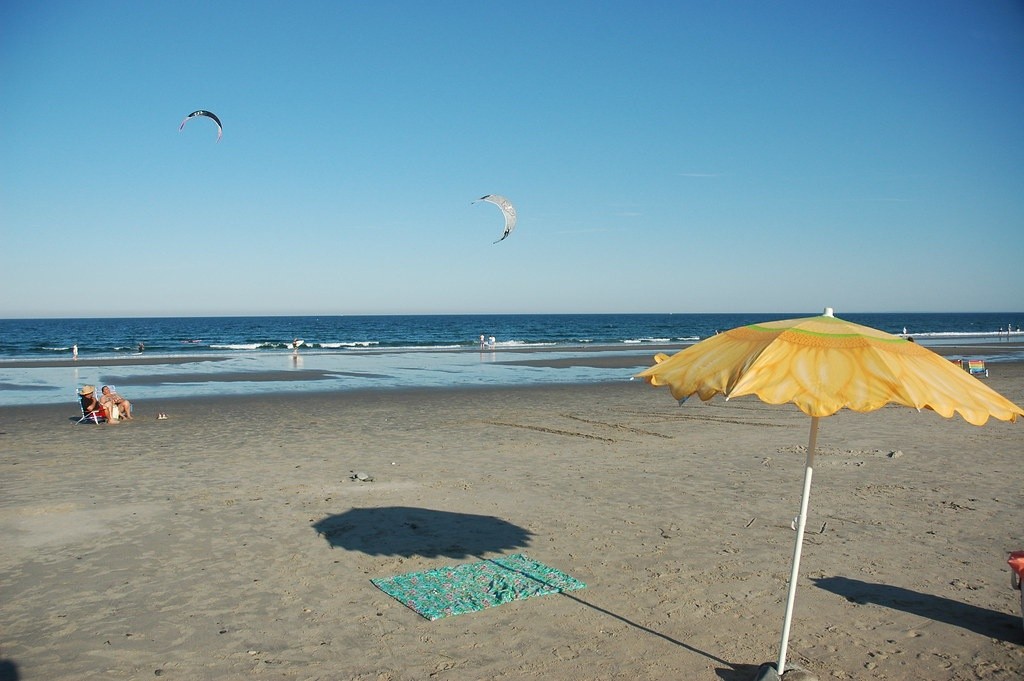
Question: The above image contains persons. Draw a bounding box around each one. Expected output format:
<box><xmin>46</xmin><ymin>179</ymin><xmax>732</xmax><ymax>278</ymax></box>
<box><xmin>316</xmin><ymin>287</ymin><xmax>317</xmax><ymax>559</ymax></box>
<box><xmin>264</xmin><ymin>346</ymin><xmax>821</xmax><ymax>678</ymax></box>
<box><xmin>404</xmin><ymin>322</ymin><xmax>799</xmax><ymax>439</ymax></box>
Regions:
<box><xmin>73</xmin><ymin>344</ymin><xmax>78</xmax><ymax>358</ymax></box>
<box><xmin>480</xmin><ymin>333</ymin><xmax>484</xmax><ymax>347</ymax></box>
<box><xmin>1007</xmin><ymin>323</ymin><xmax>1011</xmax><ymax>334</ymax></box>
<box><xmin>488</xmin><ymin>335</ymin><xmax>495</xmax><ymax>349</ymax></box>
<box><xmin>100</xmin><ymin>386</ymin><xmax>133</xmax><ymax>420</ymax></box>
<box><xmin>80</xmin><ymin>385</ymin><xmax>120</xmax><ymax>424</ymax></box>
<box><xmin>139</xmin><ymin>342</ymin><xmax>144</xmax><ymax>349</ymax></box>
<box><xmin>902</xmin><ymin>327</ymin><xmax>907</xmax><ymax>334</ymax></box>
<box><xmin>999</xmin><ymin>326</ymin><xmax>1002</xmax><ymax>334</ymax></box>
<box><xmin>1016</xmin><ymin>324</ymin><xmax>1020</xmax><ymax>332</ymax></box>
<box><xmin>715</xmin><ymin>330</ymin><xmax>718</xmax><ymax>335</ymax></box>
<box><xmin>293</xmin><ymin>337</ymin><xmax>298</xmax><ymax>353</ymax></box>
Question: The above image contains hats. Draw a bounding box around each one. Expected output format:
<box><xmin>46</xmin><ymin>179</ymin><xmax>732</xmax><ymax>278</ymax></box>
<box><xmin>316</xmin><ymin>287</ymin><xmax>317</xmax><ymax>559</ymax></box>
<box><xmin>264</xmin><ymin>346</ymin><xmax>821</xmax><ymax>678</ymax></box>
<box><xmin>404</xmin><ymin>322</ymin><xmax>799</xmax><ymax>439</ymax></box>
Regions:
<box><xmin>79</xmin><ymin>385</ymin><xmax>94</xmax><ymax>395</ymax></box>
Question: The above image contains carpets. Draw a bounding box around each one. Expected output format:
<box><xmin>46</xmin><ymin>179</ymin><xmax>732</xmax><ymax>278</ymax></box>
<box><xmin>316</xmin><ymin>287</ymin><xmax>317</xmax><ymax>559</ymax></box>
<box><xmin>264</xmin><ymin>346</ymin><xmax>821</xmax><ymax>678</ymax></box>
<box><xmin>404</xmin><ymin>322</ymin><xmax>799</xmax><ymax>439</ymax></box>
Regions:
<box><xmin>371</xmin><ymin>554</ymin><xmax>586</xmax><ymax>621</ymax></box>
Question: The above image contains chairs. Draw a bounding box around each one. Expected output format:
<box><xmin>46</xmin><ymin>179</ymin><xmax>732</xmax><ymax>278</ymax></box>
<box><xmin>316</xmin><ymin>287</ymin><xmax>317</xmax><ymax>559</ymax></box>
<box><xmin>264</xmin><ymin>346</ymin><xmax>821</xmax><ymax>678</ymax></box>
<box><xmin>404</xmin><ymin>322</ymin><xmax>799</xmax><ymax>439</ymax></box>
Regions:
<box><xmin>949</xmin><ymin>358</ymin><xmax>966</xmax><ymax>372</ymax></box>
<box><xmin>967</xmin><ymin>360</ymin><xmax>988</xmax><ymax>379</ymax></box>
<box><xmin>76</xmin><ymin>385</ymin><xmax>118</xmax><ymax>425</ymax></box>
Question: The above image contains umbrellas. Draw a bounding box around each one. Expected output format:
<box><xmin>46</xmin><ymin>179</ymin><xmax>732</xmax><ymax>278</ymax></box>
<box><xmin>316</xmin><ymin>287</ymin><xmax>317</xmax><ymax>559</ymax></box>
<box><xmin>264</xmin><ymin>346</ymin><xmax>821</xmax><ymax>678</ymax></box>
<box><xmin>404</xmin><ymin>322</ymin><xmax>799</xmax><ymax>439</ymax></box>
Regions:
<box><xmin>630</xmin><ymin>307</ymin><xmax>1024</xmax><ymax>675</ymax></box>
